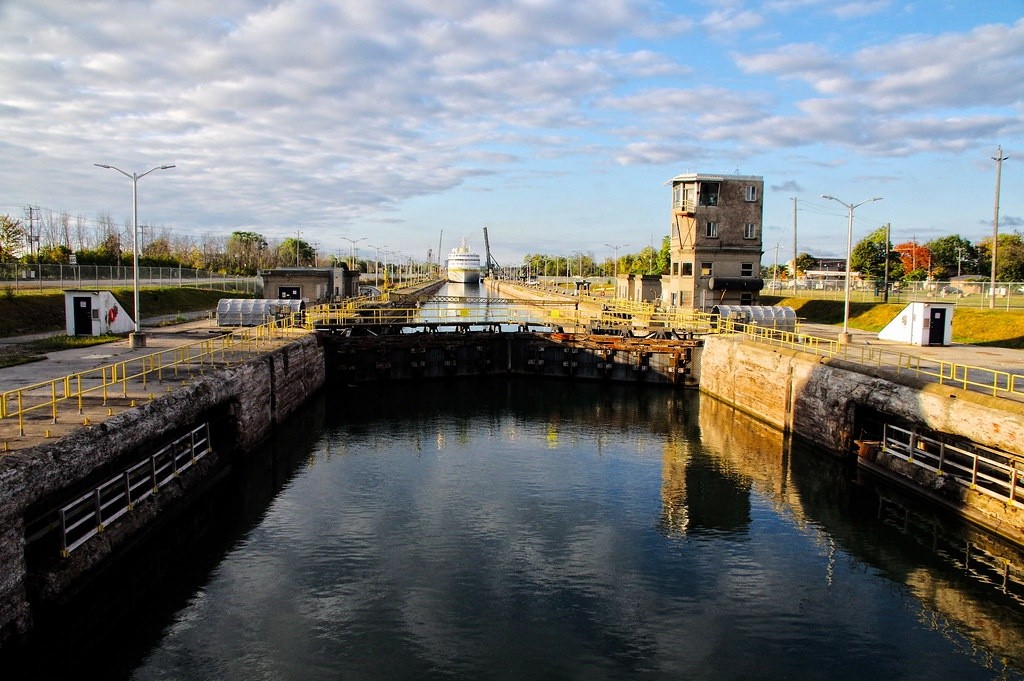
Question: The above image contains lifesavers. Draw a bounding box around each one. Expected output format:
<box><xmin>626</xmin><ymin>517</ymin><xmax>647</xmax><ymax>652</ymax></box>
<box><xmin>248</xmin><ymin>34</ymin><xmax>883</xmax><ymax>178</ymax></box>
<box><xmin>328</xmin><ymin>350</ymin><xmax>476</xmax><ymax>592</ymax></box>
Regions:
<box><xmin>109</xmin><ymin>308</ymin><xmax>117</xmax><ymax>322</ymax></box>
<box><xmin>600</xmin><ymin>291</ymin><xmax>605</xmax><ymax>297</ymax></box>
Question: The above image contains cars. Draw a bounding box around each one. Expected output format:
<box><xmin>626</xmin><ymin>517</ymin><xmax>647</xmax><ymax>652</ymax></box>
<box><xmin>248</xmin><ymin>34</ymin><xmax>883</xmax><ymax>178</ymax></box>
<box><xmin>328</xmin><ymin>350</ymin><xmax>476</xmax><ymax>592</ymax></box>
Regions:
<box><xmin>941</xmin><ymin>286</ymin><xmax>962</xmax><ymax>294</ymax></box>
<box><xmin>769</xmin><ymin>281</ymin><xmax>783</xmax><ymax>290</ymax></box>
<box><xmin>788</xmin><ymin>279</ymin><xmax>811</xmax><ymax>290</ymax></box>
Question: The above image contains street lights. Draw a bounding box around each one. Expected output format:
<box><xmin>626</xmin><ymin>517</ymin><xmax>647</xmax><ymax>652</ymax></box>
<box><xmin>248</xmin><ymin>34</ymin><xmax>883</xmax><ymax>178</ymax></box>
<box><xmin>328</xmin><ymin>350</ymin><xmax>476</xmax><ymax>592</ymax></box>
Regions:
<box><xmin>821</xmin><ymin>194</ymin><xmax>883</xmax><ymax>343</ymax></box>
<box><xmin>604</xmin><ymin>243</ymin><xmax>630</xmax><ymax>299</ymax></box>
<box><xmin>342</xmin><ymin>237</ymin><xmax>367</xmax><ymax>270</ymax></box>
<box><xmin>94</xmin><ymin>163</ymin><xmax>176</xmax><ymax>347</ymax></box>
<box><xmin>380</xmin><ymin>250</ymin><xmax>425</xmax><ymax>287</ymax></box>
<box><xmin>572</xmin><ymin>250</ymin><xmax>590</xmax><ymax>281</ymax></box>
<box><xmin>501</xmin><ymin>253</ymin><xmax>577</xmax><ymax>293</ymax></box>
<box><xmin>367</xmin><ymin>245</ymin><xmax>388</xmax><ymax>288</ymax></box>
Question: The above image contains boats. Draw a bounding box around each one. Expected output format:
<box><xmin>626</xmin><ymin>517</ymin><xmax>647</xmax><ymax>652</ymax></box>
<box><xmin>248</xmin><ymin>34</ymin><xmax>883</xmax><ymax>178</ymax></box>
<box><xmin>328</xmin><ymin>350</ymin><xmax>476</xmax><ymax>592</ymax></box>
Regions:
<box><xmin>448</xmin><ymin>238</ymin><xmax>480</xmax><ymax>282</ymax></box>
